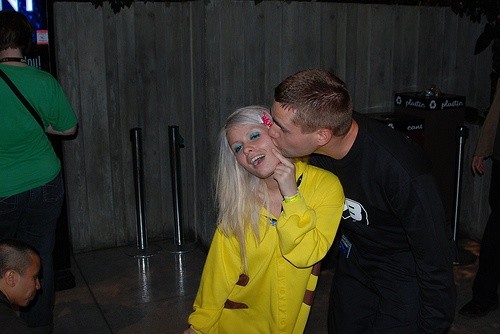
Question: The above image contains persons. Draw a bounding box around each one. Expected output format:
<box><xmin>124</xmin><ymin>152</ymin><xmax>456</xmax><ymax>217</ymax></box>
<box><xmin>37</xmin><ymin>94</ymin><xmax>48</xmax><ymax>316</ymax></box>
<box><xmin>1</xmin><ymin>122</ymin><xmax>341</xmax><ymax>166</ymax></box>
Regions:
<box><xmin>187</xmin><ymin>106</ymin><xmax>345</xmax><ymax>334</ymax></box>
<box><xmin>0</xmin><ymin>239</ymin><xmax>41</xmax><ymax>334</ymax></box>
<box><xmin>460</xmin><ymin>78</ymin><xmax>500</xmax><ymax>320</ymax></box>
<box><xmin>0</xmin><ymin>9</ymin><xmax>79</xmax><ymax>334</ymax></box>
<box><xmin>268</xmin><ymin>69</ymin><xmax>456</xmax><ymax>334</ymax></box>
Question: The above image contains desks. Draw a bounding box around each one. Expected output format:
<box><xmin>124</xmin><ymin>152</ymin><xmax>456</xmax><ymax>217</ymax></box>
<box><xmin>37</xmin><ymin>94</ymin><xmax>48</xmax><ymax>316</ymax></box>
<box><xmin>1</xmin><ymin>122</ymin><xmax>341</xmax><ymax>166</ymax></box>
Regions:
<box><xmin>359</xmin><ymin>92</ymin><xmax>467</xmax><ymax>232</ymax></box>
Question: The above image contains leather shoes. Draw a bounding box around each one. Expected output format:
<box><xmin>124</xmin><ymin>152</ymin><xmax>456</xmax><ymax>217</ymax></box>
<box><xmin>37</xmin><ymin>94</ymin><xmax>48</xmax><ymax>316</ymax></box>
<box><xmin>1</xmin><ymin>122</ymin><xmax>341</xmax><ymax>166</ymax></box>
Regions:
<box><xmin>459</xmin><ymin>299</ymin><xmax>496</xmax><ymax>316</ymax></box>
